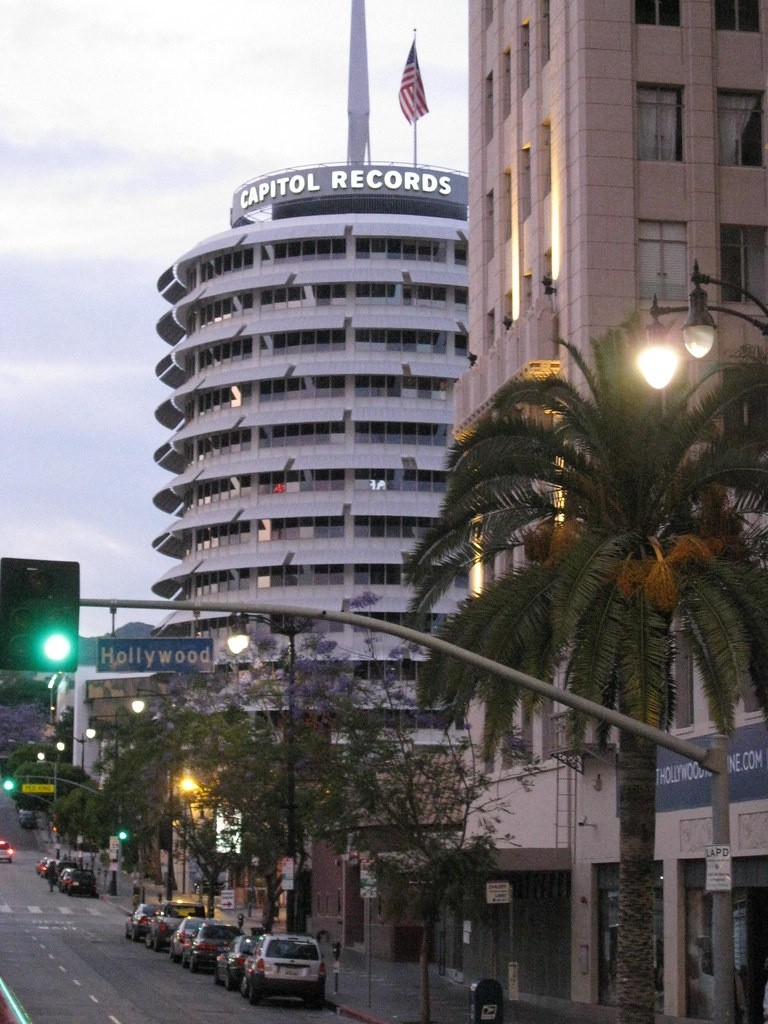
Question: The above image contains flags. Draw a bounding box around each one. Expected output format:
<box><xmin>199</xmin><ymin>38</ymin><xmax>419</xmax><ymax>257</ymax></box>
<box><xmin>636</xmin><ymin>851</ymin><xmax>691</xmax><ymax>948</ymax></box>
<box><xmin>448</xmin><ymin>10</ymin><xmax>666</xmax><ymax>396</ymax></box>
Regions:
<box><xmin>397</xmin><ymin>38</ymin><xmax>430</xmax><ymax>127</ymax></box>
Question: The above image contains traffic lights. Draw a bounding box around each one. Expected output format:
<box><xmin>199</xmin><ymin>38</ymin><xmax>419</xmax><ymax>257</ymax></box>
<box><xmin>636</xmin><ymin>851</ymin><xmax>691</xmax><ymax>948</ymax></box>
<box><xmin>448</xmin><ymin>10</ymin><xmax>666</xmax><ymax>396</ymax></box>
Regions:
<box><xmin>118</xmin><ymin>832</ymin><xmax>129</xmax><ymax>843</ymax></box>
<box><xmin>2</xmin><ymin>775</ymin><xmax>18</xmax><ymax>793</ymax></box>
<box><xmin>0</xmin><ymin>558</ymin><xmax>79</xmax><ymax>673</ymax></box>
<box><xmin>53</xmin><ymin>826</ymin><xmax>58</xmax><ymax>832</ymax></box>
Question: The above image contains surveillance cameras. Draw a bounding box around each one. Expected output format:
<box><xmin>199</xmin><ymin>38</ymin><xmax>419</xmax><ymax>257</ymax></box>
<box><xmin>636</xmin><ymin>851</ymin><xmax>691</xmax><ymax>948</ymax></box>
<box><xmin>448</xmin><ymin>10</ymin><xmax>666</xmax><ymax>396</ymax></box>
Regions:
<box><xmin>578</xmin><ymin>816</ymin><xmax>587</xmax><ymax>825</ymax></box>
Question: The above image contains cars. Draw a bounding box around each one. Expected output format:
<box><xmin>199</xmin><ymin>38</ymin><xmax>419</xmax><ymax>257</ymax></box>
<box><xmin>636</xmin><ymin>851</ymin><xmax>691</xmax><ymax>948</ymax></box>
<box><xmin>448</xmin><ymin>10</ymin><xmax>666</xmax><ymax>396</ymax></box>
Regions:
<box><xmin>37</xmin><ymin>857</ymin><xmax>97</xmax><ymax>898</ymax></box>
<box><xmin>170</xmin><ymin>917</ymin><xmax>223</xmax><ymax>964</ymax></box>
<box><xmin>215</xmin><ymin>935</ymin><xmax>259</xmax><ymax>992</ymax></box>
<box><xmin>125</xmin><ymin>903</ymin><xmax>166</xmax><ymax>942</ymax></box>
<box><xmin>19</xmin><ymin>809</ymin><xmax>37</xmax><ymax>829</ymax></box>
<box><xmin>183</xmin><ymin>922</ymin><xmax>245</xmax><ymax>973</ymax></box>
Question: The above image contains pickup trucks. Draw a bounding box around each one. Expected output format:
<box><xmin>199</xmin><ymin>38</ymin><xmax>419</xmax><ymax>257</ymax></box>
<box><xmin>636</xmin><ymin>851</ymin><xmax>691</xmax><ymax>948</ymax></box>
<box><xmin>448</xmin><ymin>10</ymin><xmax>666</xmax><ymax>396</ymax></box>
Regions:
<box><xmin>145</xmin><ymin>902</ymin><xmax>221</xmax><ymax>953</ymax></box>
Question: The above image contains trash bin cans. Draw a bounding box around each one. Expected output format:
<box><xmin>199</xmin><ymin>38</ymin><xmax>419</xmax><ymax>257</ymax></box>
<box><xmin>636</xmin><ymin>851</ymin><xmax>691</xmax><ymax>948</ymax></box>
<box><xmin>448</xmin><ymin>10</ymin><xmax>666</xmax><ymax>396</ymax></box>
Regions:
<box><xmin>470</xmin><ymin>977</ymin><xmax>503</xmax><ymax>1024</ymax></box>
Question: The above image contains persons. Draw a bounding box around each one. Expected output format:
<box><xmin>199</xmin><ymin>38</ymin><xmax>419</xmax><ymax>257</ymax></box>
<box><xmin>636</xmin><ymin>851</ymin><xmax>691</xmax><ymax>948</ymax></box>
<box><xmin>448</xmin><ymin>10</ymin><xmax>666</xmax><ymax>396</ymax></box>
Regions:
<box><xmin>44</xmin><ymin>861</ymin><xmax>59</xmax><ymax>892</ymax></box>
<box><xmin>761</xmin><ymin>956</ymin><xmax>768</xmax><ymax>1023</ymax></box>
<box><xmin>131</xmin><ymin>892</ymin><xmax>141</xmax><ymax>914</ymax></box>
<box><xmin>157</xmin><ymin>892</ymin><xmax>168</xmax><ymax>906</ymax></box>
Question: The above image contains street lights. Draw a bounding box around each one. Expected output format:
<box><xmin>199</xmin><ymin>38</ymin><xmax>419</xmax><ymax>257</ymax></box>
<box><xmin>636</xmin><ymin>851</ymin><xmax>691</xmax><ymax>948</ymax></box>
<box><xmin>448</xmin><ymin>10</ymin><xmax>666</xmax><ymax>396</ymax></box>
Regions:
<box><xmin>226</xmin><ymin>614</ymin><xmax>303</xmax><ymax>933</ymax></box>
<box><xmin>57</xmin><ymin>733</ymin><xmax>86</xmax><ymax>770</ymax></box>
<box><xmin>37</xmin><ymin>751</ymin><xmax>56</xmax><ymax>800</ymax></box>
<box><xmin>132</xmin><ymin>687</ymin><xmax>174</xmax><ymax>901</ymax></box>
<box><xmin>86</xmin><ymin>717</ymin><xmax>122</xmax><ymax>836</ymax></box>
<box><xmin>179</xmin><ymin>776</ymin><xmax>200</xmax><ymax>893</ymax></box>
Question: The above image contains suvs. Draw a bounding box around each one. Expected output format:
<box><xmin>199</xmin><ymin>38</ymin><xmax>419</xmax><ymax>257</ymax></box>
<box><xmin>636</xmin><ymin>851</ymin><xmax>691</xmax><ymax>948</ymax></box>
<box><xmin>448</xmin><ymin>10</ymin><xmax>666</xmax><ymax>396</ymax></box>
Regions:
<box><xmin>241</xmin><ymin>934</ymin><xmax>326</xmax><ymax>1010</ymax></box>
<box><xmin>0</xmin><ymin>841</ymin><xmax>11</xmax><ymax>863</ymax></box>
